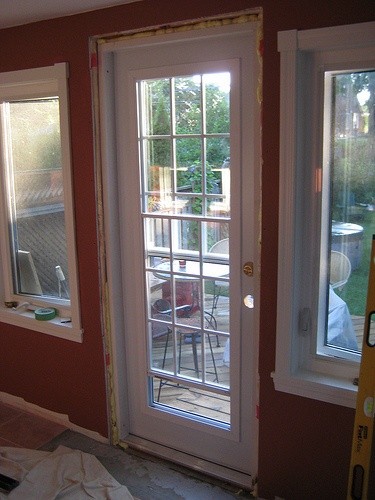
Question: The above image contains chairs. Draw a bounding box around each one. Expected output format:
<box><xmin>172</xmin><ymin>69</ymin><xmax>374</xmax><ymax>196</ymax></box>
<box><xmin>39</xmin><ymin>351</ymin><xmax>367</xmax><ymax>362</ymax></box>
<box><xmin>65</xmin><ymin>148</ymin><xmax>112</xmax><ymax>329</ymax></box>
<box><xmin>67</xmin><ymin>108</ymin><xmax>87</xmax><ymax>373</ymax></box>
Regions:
<box><xmin>149</xmin><ymin>304</ymin><xmax>193</xmax><ymax>341</ymax></box>
<box><xmin>208</xmin><ymin>238</ymin><xmax>230</xmax><ymax>325</ymax></box>
<box><xmin>329</xmin><ymin>251</ymin><xmax>352</xmax><ymax>296</ymax></box>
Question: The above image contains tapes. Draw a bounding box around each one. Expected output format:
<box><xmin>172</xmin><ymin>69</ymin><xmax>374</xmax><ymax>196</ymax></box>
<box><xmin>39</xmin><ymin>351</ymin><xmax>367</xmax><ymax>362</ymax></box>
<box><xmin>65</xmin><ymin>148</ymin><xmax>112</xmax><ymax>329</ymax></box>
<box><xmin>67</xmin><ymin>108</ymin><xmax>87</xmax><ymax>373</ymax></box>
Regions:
<box><xmin>34</xmin><ymin>307</ymin><xmax>56</xmax><ymax>320</ymax></box>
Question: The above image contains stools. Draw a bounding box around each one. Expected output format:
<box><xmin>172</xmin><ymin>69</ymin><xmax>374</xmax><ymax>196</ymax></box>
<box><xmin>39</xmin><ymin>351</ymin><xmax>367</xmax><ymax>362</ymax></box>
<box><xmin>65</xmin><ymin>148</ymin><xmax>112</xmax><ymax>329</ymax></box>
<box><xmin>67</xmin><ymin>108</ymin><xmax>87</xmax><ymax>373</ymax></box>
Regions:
<box><xmin>158</xmin><ymin>319</ymin><xmax>220</xmax><ymax>403</ymax></box>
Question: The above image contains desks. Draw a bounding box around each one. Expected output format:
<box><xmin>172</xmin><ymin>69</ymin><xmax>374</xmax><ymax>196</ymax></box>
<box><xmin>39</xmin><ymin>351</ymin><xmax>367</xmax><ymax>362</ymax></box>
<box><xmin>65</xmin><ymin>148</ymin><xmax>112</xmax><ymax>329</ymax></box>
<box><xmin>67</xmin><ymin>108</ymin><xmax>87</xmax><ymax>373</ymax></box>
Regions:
<box><xmin>153</xmin><ymin>260</ymin><xmax>230</xmax><ymax>347</ymax></box>
